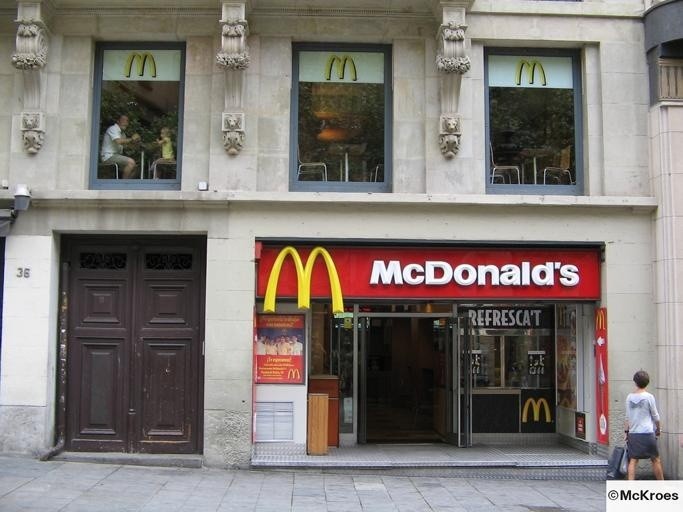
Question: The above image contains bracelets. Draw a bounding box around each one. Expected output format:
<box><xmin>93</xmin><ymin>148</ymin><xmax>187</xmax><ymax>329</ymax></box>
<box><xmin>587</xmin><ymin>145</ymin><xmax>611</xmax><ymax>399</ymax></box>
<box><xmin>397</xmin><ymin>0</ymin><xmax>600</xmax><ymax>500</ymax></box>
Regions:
<box><xmin>623</xmin><ymin>429</ymin><xmax>628</xmax><ymax>433</ymax></box>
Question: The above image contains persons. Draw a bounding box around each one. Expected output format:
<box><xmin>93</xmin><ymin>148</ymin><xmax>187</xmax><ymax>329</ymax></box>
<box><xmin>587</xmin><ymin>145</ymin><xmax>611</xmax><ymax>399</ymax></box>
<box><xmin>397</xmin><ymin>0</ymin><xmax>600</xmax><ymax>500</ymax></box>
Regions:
<box><xmin>148</xmin><ymin>127</ymin><xmax>173</xmax><ymax>171</ymax></box>
<box><xmin>99</xmin><ymin>113</ymin><xmax>141</xmax><ymax>179</ymax></box>
<box><xmin>622</xmin><ymin>369</ymin><xmax>665</xmax><ymax>479</ymax></box>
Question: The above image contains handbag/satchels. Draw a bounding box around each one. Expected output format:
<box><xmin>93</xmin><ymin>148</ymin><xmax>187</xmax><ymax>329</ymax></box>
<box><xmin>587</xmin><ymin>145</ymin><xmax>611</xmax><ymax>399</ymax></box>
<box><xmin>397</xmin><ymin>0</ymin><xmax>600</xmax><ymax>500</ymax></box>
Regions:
<box><xmin>606</xmin><ymin>444</ymin><xmax>624</xmax><ymax>478</ymax></box>
<box><xmin>619</xmin><ymin>449</ymin><xmax>628</xmax><ymax>474</ymax></box>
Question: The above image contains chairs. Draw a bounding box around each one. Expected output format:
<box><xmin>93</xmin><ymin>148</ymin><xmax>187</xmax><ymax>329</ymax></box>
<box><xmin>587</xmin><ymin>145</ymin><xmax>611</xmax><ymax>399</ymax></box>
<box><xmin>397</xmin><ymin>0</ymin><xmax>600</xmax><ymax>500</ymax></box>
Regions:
<box><xmin>488</xmin><ymin>140</ymin><xmax>574</xmax><ymax>186</ymax></box>
<box><xmin>294</xmin><ymin>142</ymin><xmax>328</xmax><ymax>183</ymax></box>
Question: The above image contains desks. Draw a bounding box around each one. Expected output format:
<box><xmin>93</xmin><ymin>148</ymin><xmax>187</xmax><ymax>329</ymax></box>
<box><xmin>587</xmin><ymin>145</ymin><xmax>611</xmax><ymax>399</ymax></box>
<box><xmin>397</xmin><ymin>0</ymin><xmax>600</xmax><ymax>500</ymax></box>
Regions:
<box><xmin>328</xmin><ymin>141</ymin><xmax>370</xmax><ymax>182</ymax></box>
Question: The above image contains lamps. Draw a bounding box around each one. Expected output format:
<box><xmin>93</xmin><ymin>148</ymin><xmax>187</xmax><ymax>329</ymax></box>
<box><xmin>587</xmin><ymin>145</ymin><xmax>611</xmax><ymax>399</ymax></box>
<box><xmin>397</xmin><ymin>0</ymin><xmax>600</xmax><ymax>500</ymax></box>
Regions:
<box><xmin>14</xmin><ymin>182</ymin><xmax>31</xmax><ymax>212</ymax></box>
